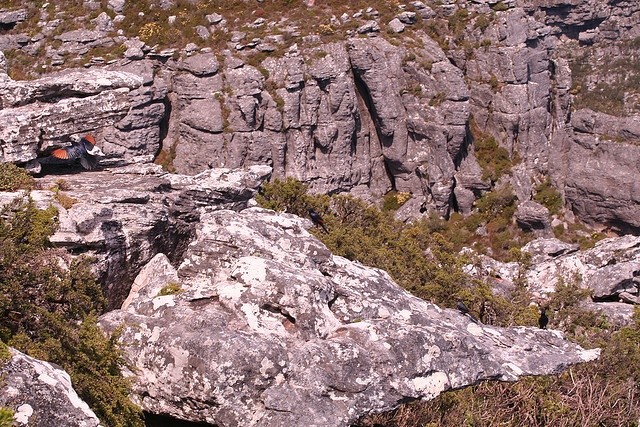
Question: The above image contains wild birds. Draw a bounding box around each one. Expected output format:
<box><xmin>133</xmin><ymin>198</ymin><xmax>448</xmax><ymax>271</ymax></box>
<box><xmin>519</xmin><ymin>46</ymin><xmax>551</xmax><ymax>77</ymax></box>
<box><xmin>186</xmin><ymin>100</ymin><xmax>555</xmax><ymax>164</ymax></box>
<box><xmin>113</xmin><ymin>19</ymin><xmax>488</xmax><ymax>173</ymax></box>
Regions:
<box><xmin>306</xmin><ymin>207</ymin><xmax>329</xmax><ymax>235</ymax></box>
<box><xmin>456</xmin><ymin>299</ymin><xmax>479</xmax><ymax>324</ymax></box>
<box><xmin>37</xmin><ymin>133</ymin><xmax>100</xmax><ymax>173</ymax></box>
<box><xmin>537</xmin><ymin>310</ymin><xmax>549</xmax><ymax>330</ymax></box>
